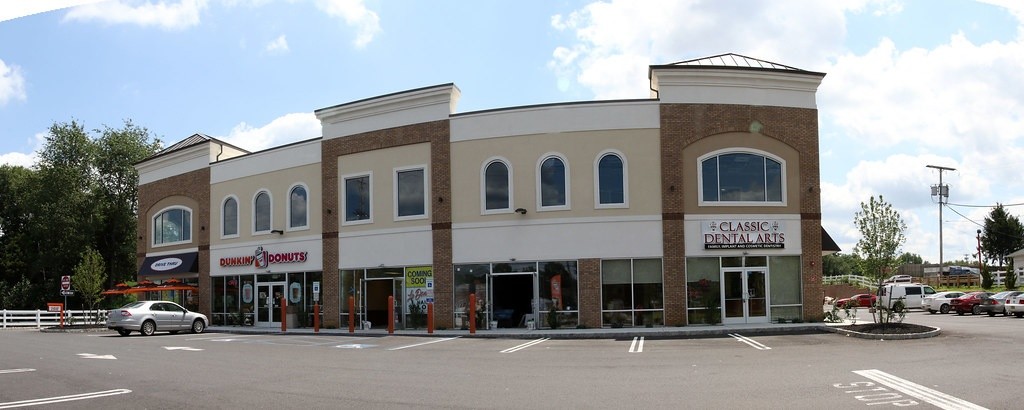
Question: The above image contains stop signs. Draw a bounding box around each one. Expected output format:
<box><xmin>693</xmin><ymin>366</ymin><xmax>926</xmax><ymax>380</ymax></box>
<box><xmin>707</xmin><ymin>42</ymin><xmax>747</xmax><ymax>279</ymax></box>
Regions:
<box><xmin>61</xmin><ymin>276</ymin><xmax>71</xmax><ymax>290</ymax></box>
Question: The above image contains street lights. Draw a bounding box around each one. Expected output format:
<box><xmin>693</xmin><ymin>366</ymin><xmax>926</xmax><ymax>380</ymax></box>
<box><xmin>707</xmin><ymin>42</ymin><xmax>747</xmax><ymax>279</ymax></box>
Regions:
<box><xmin>977</xmin><ymin>229</ymin><xmax>982</xmax><ymax>274</ymax></box>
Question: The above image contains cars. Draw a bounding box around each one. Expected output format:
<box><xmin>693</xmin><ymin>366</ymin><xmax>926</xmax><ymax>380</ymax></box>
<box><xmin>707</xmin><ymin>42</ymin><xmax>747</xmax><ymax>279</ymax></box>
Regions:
<box><xmin>979</xmin><ymin>291</ymin><xmax>1024</xmax><ymax>317</ymax></box>
<box><xmin>106</xmin><ymin>300</ymin><xmax>209</xmax><ymax>336</ymax></box>
<box><xmin>833</xmin><ymin>293</ymin><xmax>877</xmax><ymax>309</ymax></box>
<box><xmin>1004</xmin><ymin>293</ymin><xmax>1024</xmax><ymax>318</ymax></box>
<box><xmin>949</xmin><ymin>292</ymin><xmax>997</xmax><ymax>315</ymax></box>
<box><xmin>890</xmin><ymin>274</ymin><xmax>913</xmax><ymax>280</ymax></box>
<box><xmin>921</xmin><ymin>292</ymin><xmax>966</xmax><ymax>314</ymax></box>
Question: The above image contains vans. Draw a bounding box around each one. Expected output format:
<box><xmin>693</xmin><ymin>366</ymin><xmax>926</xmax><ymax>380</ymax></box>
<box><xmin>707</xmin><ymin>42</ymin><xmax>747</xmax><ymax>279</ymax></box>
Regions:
<box><xmin>876</xmin><ymin>283</ymin><xmax>937</xmax><ymax>312</ymax></box>
